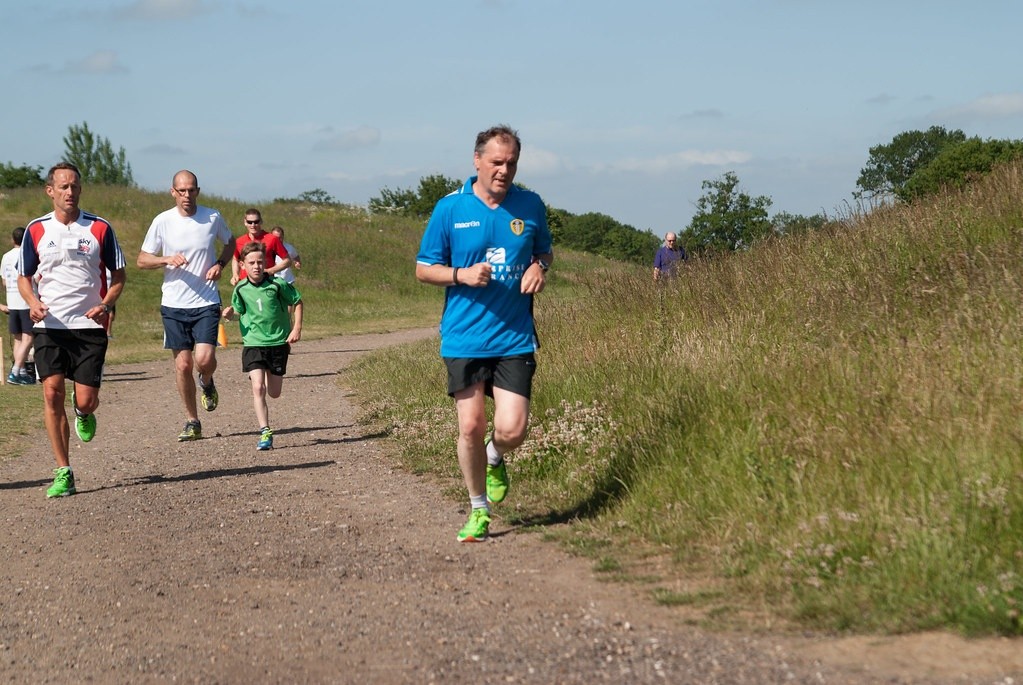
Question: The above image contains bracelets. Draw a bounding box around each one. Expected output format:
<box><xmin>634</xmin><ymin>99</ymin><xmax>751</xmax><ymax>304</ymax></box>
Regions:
<box><xmin>98</xmin><ymin>304</ymin><xmax>111</xmax><ymax>313</ymax></box>
<box><xmin>216</xmin><ymin>260</ymin><xmax>226</xmax><ymax>268</ymax></box>
<box><xmin>453</xmin><ymin>267</ymin><xmax>460</xmax><ymax>285</ymax></box>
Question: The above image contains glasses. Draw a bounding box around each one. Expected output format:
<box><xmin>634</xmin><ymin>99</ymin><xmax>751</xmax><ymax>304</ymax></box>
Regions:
<box><xmin>244</xmin><ymin>218</ymin><xmax>262</xmax><ymax>224</ymax></box>
<box><xmin>173</xmin><ymin>186</ymin><xmax>198</xmax><ymax>196</ymax></box>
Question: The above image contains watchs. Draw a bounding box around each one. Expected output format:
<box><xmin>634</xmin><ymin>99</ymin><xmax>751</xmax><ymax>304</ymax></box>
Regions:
<box><xmin>535</xmin><ymin>258</ymin><xmax>549</xmax><ymax>272</ymax></box>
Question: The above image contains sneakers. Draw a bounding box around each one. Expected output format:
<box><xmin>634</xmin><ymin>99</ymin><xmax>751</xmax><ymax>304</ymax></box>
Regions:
<box><xmin>456</xmin><ymin>505</ymin><xmax>491</xmax><ymax>542</ymax></box>
<box><xmin>177</xmin><ymin>418</ymin><xmax>202</xmax><ymax>440</ymax></box>
<box><xmin>72</xmin><ymin>391</ymin><xmax>96</xmax><ymax>442</ymax></box>
<box><xmin>484</xmin><ymin>435</ymin><xmax>507</xmax><ymax>504</ymax></box>
<box><xmin>256</xmin><ymin>429</ymin><xmax>274</xmax><ymax>451</ymax></box>
<box><xmin>47</xmin><ymin>464</ymin><xmax>77</xmax><ymax>498</ymax></box>
<box><xmin>19</xmin><ymin>371</ymin><xmax>35</xmax><ymax>384</ymax></box>
<box><xmin>6</xmin><ymin>373</ymin><xmax>27</xmax><ymax>386</ymax></box>
<box><xmin>198</xmin><ymin>371</ymin><xmax>218</xmax><ymax>412</ymax></box>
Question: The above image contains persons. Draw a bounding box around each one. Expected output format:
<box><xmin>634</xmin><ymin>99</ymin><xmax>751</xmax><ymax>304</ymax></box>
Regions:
<box><xmin>1</xmin><ymin>227</ymin><xmax>41</xmax><ymax>384</ymax></box>
<box><xmin>415</xmin><ymin>121</ymin><xmax>552</xmax><ymax>542</ymax></box>
<box><xmin>271</xmin><ymin>226</ymin><xmax>302</xmax><ymax>330</ymax></box>
<box><xmin>221</xmin><ymin>241</ymin><xmax>303</xmax><ymax>451</ymax></box>
<box><xmin>105</xmin><ymin>266</ymin><xmax>116</xmax><ymax>339</ymax></box>
<box><xmin>15</xmin><ymin>162</ymin><xmax>127</xmax><ymax>498</ymax></box>
<box><xmin>229</xmin><ymin>208</ymin><xmax>292</xmax><ymax>286</ymax></box>
<box><xmin>137</xmin><ymin>169</ymin><xmax>237</xmax><ymax>441</ymax></box>
<box><xmin>654</xmin><ymin>231</ymin><xmax>688</xmax><ymax>314</ymax></box>
